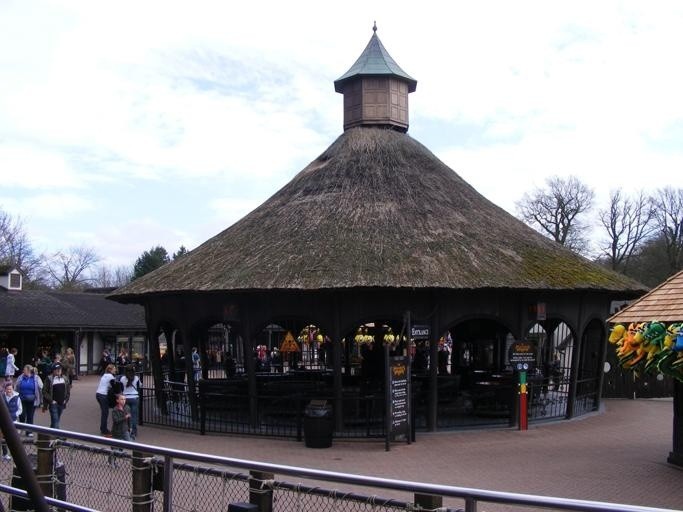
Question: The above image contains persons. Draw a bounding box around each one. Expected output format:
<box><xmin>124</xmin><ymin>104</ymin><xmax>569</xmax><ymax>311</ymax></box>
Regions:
<box><xmin>94</xmin><ymin>364</ymin><xmax>117</xmax><ymax>437</ymax></box>
<box><xmin>40</xmin><ymin>362</ymin><xmax>72</xmax><ymax>441</ymax></box>
<box><xmin>13</xmin><ymin>364</ymin><xmax>44</xmax><ymax>438</ymax></box>
<box><xmin>552</xmin><ymin>354</ymin><xmax>561</xmax><ymax>390</ymax></box>
<box><xmin>0</xmin><ymin>382</ymin><xmax>23</xmax><ymax>462</ymax></box>
<box><xmin>107</xmin><ymin>394</ymin><xmax>132</xmax><ymax>469</ymax></box>
<box><xmin>5</xmin><ymin>344</ymin><xmax>149</xmax><ymax>389</ymax></box>
<box><xmin>192</xmin><ymin>348</ymin><xmax>201</xmax><ymax>382</ymax></box>
<box><xmin>120</xmin><ymin>364</ymin><xmax>142</xmax><ymax>437</ymax></box>
<box><xmin>256</xmin><ymin>339</ymin><xmax>297</xmax><ymax>368</ymax></box>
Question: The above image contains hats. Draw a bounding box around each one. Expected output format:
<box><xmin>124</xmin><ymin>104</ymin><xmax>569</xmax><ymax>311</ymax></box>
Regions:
<box><xmin>52</xmin><ymin>364</ymin><xmax>63</xmax><ymax>369</ymax></box>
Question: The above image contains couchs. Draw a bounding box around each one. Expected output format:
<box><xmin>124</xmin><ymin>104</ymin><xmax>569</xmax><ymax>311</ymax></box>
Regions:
<box><xmin>198</xmin><ymin>378</ymin><xmax>247</xmax><ymax>411</ymax></box>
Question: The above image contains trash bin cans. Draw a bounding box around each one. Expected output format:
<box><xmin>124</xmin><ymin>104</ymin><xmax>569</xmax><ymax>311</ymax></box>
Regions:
<box><xmin>11</xmin><ymin>461</ymin><xmax>66</xmax><ymax>512</ymax></box>
<box><xmin>302</xmin><ymin>399</ymin><xmax>333</xmax><ymax>448</ymax></box>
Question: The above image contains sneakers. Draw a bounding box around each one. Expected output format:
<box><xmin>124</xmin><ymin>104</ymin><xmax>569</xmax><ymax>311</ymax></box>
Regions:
<box><xmin>2</xmin><ymin>454</ymin><xmax>10</xmax><ymax>459</ymax></box>
<box><xmin>18</xmin><ymin>428</ymin><xmax>34</xmax><ymax>436</ymax></box>
